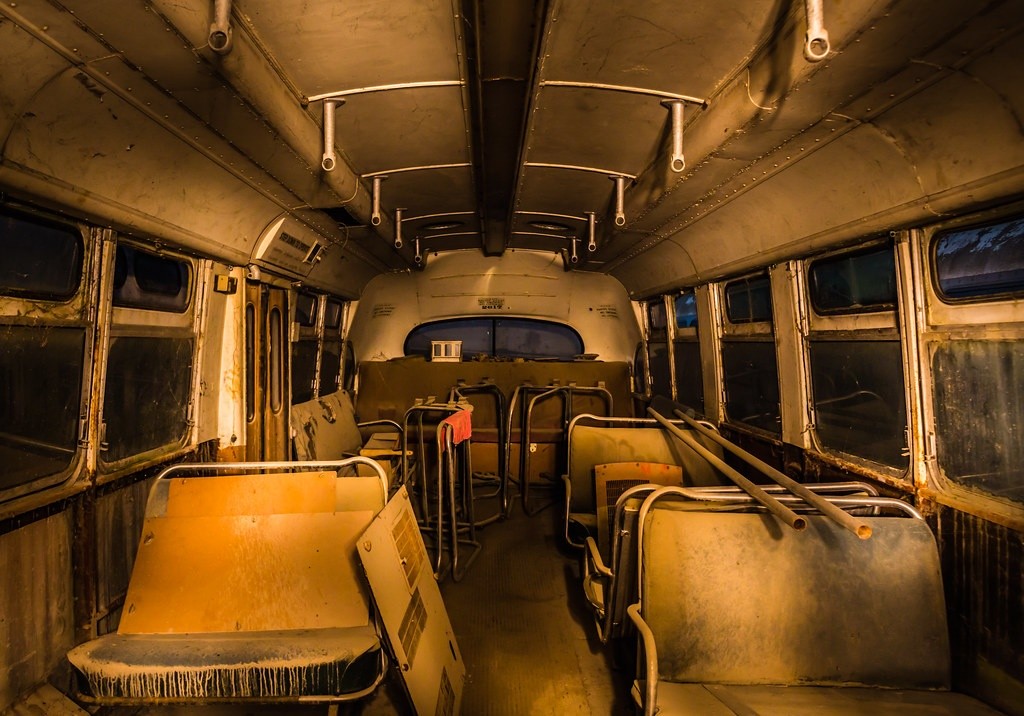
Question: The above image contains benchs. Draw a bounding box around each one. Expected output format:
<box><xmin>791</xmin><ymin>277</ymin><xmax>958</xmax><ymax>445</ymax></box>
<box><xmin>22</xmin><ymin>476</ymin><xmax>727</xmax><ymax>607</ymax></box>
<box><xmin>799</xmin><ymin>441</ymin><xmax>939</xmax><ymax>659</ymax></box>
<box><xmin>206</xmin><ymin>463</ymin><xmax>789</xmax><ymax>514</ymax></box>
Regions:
<box><xmin>624</xmin><ymin>486</ymin><xmax>1004</xmax><ymax>716</ymax></box>
<box><xmin>69</xmin><ymin>456</ymin><xmax>388</xmax><ymax>704</ymax></box>
<box><xmin>290</xmin><ymin>389</ymin><xmax>413</xmax><ymax>508</ymax></box>
<box><xmin>560</xmin><ymin>412</ymin><xmax>726</xmax><ymax>551</ymax></box>
<box><xmin>579</xmin><ymin>481</ymin><xmax>885</xmax><ymax>647</ymax></box>
